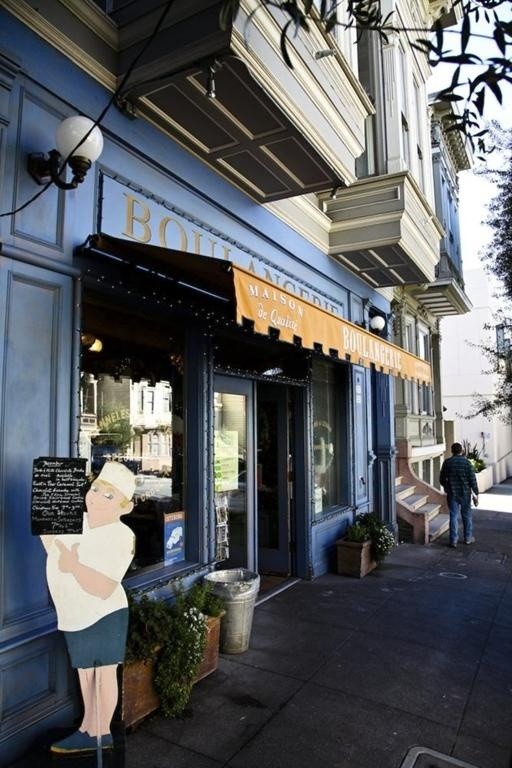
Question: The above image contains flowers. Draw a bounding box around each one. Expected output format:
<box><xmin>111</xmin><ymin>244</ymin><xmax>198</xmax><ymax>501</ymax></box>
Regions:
<box><xmin>461</xmin><ymin>440</ymin><xmax>486</xmax><ymax>472</ymax></box>
<box><xmin>343</xmin><ymin>512</ymin><xmax>395</xmax><ymax>566</ymax></box>
<box><xmin>116</xmin><ymin>576</ymin><xmax>232</xmax><ymax>718</ymax></box>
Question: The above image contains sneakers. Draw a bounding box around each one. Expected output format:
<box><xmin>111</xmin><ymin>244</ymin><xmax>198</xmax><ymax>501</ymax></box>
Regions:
<box><xmin>450</xmin><ymin>536</ymin><xmax>476</xmax><ymax>548</ymax></box>
<box><xmin>48</xmin><ymin>730</ymin><xmax>115</xmax><ymax>753</ymax></box>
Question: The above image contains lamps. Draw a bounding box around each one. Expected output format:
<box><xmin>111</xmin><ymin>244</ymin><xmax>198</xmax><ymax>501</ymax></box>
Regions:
<box><xmin>356</xmin><ymin>316</ymin><xmax>386</xmax><ymax>335</ymax></box>
<box><xmin>28</xmin><ymin>117</ymin><xmax>104</xmax><ymax>191</ymax></box>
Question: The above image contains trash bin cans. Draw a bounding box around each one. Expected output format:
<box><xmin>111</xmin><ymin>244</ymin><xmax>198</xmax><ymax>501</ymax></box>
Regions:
<box><xmin>203</xmin><ymin>568</ymin><xmax>260</xmax><ymax>655</ymax></box>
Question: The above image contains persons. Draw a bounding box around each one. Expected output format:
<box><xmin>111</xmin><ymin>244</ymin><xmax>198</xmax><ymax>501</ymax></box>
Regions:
<box><xmin>39</xmin><ymin>456</ymin><xmax>139</xmax><ymax>755</ymax></box>
<box><xmin>437</xmin><ymin>442</ymin><xmax>481</xmax><ymax>549</ymax></box>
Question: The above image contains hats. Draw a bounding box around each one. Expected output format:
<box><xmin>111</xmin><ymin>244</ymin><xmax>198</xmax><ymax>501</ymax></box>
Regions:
<box><xmin>99</xmin><ymin>460</ymin><xmax>137</xmax><ymax>500</ymax></box>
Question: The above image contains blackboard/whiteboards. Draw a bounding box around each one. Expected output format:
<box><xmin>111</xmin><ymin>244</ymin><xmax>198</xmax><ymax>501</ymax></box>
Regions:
<box><xmin>31</xmin><ymin>456</ymin><xmax>88</xmax><ymax>535</ymax></box>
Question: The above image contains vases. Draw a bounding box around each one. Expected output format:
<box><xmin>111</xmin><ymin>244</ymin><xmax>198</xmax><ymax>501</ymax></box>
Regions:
<box><xmin>114</xmin><ymin>614</ymin><xmax>221</xmax><ymax>729</ymax></box>
<box><xmin>335</xmin><ymin>540</ymin><xmax>380</xmax><ymax>578</ymax></box>
<box><xmin>475</xmin><ymin>466</ymin><xmax>494</xmax><ymax>493</ymax></box>
